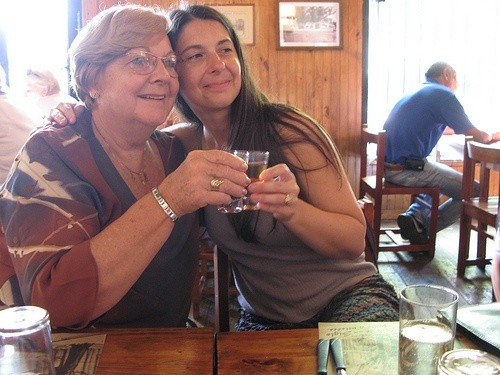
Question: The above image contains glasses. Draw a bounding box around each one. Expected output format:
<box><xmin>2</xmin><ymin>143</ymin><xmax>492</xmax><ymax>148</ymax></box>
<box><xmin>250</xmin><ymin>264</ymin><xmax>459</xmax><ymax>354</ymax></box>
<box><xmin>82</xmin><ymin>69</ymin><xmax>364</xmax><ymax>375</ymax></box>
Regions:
<box><xmin>118</xmin><ymin>52</ymin><xmax>186</xmax><ymax>77</ymax></box>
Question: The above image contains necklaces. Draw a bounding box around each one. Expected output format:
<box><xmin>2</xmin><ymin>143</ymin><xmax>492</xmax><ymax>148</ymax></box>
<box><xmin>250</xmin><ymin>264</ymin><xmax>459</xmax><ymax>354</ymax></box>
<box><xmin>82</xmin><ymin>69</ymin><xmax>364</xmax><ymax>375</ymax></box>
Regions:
<box><xmin>90</xmin><ymin>118</ymin><xmax>151</xmax><ymax>186</ymax></box>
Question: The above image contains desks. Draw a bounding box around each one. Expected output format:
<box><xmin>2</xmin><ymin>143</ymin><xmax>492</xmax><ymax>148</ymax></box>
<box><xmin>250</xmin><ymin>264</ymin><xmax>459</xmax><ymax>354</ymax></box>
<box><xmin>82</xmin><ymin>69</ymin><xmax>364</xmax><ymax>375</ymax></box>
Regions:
<box><xmin>215</xmin><ymin>322</ymin><xmax>485</xmax><ymax>375</ymax></box>
<box><xmin>436</xmin><ymin>132</ymin><xmax>500</xmax><ymax>171</ymax></box>
<box><xmin>51</xmin><ymin>327</ymin><xmax>215</xmax><ymax>375</ymax></box>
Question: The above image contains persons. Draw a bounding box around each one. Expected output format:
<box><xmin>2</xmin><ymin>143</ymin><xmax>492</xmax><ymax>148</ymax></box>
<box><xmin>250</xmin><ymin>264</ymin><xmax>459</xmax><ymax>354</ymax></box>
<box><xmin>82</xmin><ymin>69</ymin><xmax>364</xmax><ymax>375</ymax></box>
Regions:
<box><xmin>0</xmin><ymin>21</ymin><xmax>69</xmax><ymax>184</ymax></box>
<box><xmin>0</xmin><ymin>4</ymin><xmax>250</xmax><ymax>334</ymax></box>
<box><xmin>379</xmin><ymin>60</ymin><xmax>500</xmax><ymax>254</ymax></box>
<box><xmin>40</xmin><ymin>5</ymin><xmax>411</xmax><ymax>331</ymax></box>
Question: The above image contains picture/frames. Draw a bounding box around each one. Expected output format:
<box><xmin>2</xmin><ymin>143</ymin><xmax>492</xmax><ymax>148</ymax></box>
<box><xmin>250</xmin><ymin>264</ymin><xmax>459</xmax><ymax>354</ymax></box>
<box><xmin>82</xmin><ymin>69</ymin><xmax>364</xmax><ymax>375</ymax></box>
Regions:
<box><xmin>205</xmin><ymin>3</ymin><xmax>254</xmax><ymax>46</ymax></box>
<box><xmin>276</xmin><ymin>0</ymin><xmax>343</xmax><ymax>51</ymax></box>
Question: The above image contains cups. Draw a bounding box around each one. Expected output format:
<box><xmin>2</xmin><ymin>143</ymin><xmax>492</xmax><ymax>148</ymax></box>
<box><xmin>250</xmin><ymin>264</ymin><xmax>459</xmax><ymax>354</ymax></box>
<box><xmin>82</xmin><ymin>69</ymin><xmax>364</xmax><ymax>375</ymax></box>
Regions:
<box><xmin>437</xmin><ymin>349</ymin><xmax>500</xmax><ymax>375</ymax></box>
<box><xmin>399</xmin><ymin>284</ymin><xmax>459</xmax><ymax>375</ymax></box>
<box><xmin>0</xmin><ymin>306</ymin><xmax>57</xmax><ymax>375</ymax></box>
<box><xmin>217</xmin><ymin>150</ymin><xmax>249</xmax><ymax>213</ymax></box>
<box><xmin>244</xmin><ymin>150</ymin><xmax>269</xmax><ymax>211</ymax></box>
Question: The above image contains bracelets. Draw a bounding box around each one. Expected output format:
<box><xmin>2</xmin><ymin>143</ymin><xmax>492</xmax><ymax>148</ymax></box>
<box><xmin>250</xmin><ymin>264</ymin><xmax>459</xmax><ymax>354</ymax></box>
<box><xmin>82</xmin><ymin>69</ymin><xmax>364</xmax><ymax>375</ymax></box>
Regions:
<box><xmin>150</xmin><ymin>186</ymin><xmax>181</xmax><ymax>222</ymax></box>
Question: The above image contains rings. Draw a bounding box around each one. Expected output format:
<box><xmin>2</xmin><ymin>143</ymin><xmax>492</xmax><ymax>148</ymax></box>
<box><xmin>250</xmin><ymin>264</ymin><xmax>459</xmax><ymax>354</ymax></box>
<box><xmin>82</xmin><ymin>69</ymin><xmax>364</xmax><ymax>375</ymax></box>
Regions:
<box><xmin>211</xmin><ymin>176</ymin><xmax>223</xmax><ymax>192</ymax></box>
<box><xmin>285</xmin><ymin>193</ymin><xmax>291</xmax><ymax>206</ymax></box>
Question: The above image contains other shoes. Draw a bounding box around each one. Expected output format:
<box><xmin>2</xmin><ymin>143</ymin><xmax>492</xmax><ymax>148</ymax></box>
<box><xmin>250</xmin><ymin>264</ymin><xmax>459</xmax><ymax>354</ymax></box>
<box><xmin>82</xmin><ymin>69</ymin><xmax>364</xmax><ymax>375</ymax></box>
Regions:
<box><xmin>398</xmin><ymin>213</ymin><xmax>428</xmax><ymax>245</ymax></box>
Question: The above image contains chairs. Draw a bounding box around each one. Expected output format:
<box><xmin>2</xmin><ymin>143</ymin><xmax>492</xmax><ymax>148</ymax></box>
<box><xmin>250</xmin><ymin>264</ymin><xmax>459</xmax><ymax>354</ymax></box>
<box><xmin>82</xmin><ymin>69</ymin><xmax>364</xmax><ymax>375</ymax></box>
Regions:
<box><xmin>457</xmin><ymin>142</ymin><xmax>500</xmax><ymax>277</ymax></box>
<box><xmin>359</xmin><ymin>124</ymin><xmax>439</xmax><ymax>261</ymax></box>
